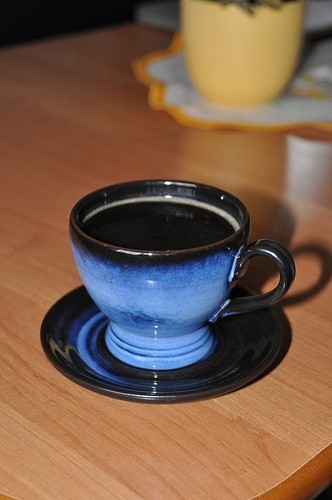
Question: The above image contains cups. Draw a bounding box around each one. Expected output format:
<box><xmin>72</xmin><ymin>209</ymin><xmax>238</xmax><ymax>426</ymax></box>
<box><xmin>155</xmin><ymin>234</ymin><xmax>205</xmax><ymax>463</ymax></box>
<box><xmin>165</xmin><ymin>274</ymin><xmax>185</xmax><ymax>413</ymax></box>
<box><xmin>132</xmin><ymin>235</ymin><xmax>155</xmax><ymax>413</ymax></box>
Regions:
<box><xmin>70</xmin><ymin>179</ymin><xmax>296</xmax><ymax>372</ymax></box>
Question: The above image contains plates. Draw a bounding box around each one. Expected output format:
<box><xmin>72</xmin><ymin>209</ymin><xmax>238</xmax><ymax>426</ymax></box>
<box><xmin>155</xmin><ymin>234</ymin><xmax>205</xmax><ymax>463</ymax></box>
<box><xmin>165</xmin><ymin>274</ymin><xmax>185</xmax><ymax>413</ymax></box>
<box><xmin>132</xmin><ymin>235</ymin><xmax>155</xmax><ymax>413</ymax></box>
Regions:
<box><xmin>40</xmin><ymin>283</ymin><xmax>292</xmax><ymax>405</ymax></box>
